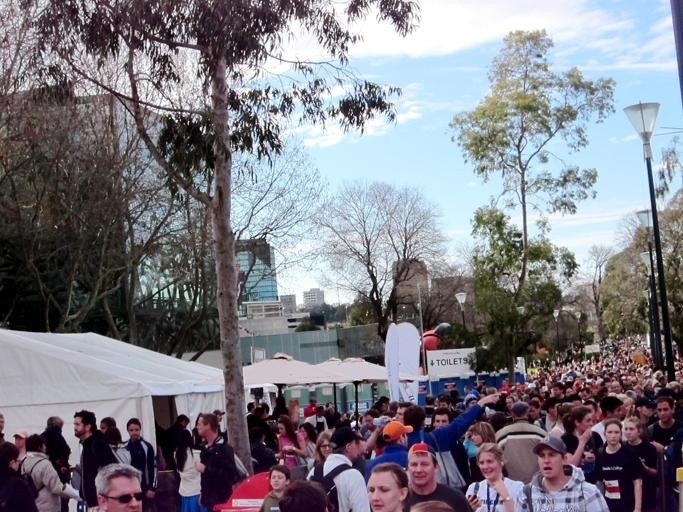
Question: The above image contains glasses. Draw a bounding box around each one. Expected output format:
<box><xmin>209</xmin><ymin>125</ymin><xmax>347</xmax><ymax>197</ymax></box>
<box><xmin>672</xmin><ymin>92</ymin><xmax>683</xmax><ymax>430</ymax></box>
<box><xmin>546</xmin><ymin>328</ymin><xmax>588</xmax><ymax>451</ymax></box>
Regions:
<box><xmin>318</xmin><ymin>443</ymin><xmax>333</xmax><ymax>448</ymax></box>
<box><xmin>99</xmin><ymin>488</ymin><xmax>144</xmax><ymax>502</ymax></box>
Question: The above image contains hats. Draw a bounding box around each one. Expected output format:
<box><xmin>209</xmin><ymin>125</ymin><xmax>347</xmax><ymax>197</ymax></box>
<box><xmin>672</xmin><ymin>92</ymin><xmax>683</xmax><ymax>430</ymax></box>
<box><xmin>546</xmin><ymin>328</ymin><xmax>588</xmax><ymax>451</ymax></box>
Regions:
<box><xmin>371</xmin><ymin>415</ymin><xmax>390</xmax><ymax>425</ymax></box>
<box><xmin>532</xmin><ymin>433</ymin><xmax>567</xmax><ymax>457</ymax></box>
<box><xmin>213</xmin><ymin>409</ymin><xmax>228</xmax><ymax>416</ymax></box>
<box><xmin>11</xmin><ymin>430</ymin><xmax>28</xmax><ymax>440</ymax></box>
<box><xmin>408</xmin><ymin>442</ymin><xmax>437</xmax><ymax>458</ymax></box>
<box><xmin>330</xmin><ymin>425</ymin><xmax>367</xmax><ymax>449</ymax></box>
<box><xmin>380</xmin><ymin>421</ymin><xmax>416</xmax><ymax>441</ymax></box>
<box><xmin>634</xmin><ymin>396</ymin><xmax>657</xmax><ymax>411</ymax></box>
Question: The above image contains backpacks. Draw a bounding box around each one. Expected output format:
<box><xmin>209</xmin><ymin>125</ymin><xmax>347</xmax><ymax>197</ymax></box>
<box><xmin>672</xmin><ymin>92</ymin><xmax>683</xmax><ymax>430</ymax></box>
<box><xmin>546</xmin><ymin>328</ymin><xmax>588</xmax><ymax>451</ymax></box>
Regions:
<box><xmin>309</xmin><ymin>474</ymin><xmax>339</xmax><ymax>512</ymax></box>
<box><xmin>20</xmin><ymin>473</ymin><xmax>38</xmax><ymax>500</ymax></box>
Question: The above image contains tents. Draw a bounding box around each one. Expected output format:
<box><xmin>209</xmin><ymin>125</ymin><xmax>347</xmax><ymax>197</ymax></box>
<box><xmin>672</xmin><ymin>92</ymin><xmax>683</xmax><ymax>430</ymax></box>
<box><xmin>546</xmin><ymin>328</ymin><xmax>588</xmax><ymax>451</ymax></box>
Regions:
<box><xmin>1</xmin><ymin>330</ymin><xmax>278</xmax><ymax>511</ymax></box>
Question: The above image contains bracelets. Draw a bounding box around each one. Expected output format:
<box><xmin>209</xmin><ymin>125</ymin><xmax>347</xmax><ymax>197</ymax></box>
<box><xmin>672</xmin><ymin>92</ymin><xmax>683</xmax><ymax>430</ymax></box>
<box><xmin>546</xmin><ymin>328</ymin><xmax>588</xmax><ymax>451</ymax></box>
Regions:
<box><xmin>503</xmin><ymin>496</ymin><xmax>513</xmax><ymax>502</ymax></box>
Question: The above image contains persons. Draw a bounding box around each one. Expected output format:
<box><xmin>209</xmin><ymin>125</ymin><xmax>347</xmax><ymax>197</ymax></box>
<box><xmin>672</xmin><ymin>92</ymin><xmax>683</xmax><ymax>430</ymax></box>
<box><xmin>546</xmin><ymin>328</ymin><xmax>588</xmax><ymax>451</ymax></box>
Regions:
<box><xmin>246</xmin><ymin>397</ymin><xmax>473</xmax><ymax>510</ymax></box>
<box><xmin>165</xmin><ymin>405</ymin><xmax>234</xmax><ymax>511</ymax></box>
<box><xmin>73</xmin><ymin>409</ymin><xmax>156</xmax><ymax>511</ymax></box>
<box><xmin>432</xmin><ymin>353</ymin><xmax>681</xmax><ymax>511</ymax></box>
<box><xmin>2</xmin><ymin>415</ymin><xmax>82</xmax><ymax>510</ymax></box>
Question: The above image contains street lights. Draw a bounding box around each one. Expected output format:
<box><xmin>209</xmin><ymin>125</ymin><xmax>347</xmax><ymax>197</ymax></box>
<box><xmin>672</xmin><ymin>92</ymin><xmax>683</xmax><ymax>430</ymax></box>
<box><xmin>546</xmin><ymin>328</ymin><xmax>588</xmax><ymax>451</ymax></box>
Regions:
<box><xmin>575</xmin><ymin>310</ymin><xmax>583</xmax><ymax>355</ymax></box>
<box><xmin>455</xmin><ymin>290</ymin><xmax>470</xmax><ymax>348</ymax></box>
<box><xmin>551</xmin><ymin>309</ymin><xmax>560</xmax><ymax>358</ymax></box>
<box><xmin>621</xmin><ymin>98</ymin><xmax>675</xmax><ymax>382</ymax></box>
<box><xmin>632</xmin><ymin>208</ymin><xmax>665</xmax><ymax>376</ymax></box>
<box><xmin>517</xmin><ymin>305</ymin><xmax>527</xmax><ymax>359</ymax></box>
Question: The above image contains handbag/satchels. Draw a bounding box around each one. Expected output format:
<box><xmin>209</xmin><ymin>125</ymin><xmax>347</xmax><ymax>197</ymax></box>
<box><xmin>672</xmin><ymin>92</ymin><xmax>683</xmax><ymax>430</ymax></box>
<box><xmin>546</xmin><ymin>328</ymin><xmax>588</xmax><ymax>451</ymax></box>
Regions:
<box><xmin>288</xmin><ymin>465</ymin><xmax>309</xmax><ymax>482</ymax></box>
<box><xmin>434</xmin><ymin>450</ymin><xmax>467</xmax><ymax>489</ymax></box>
